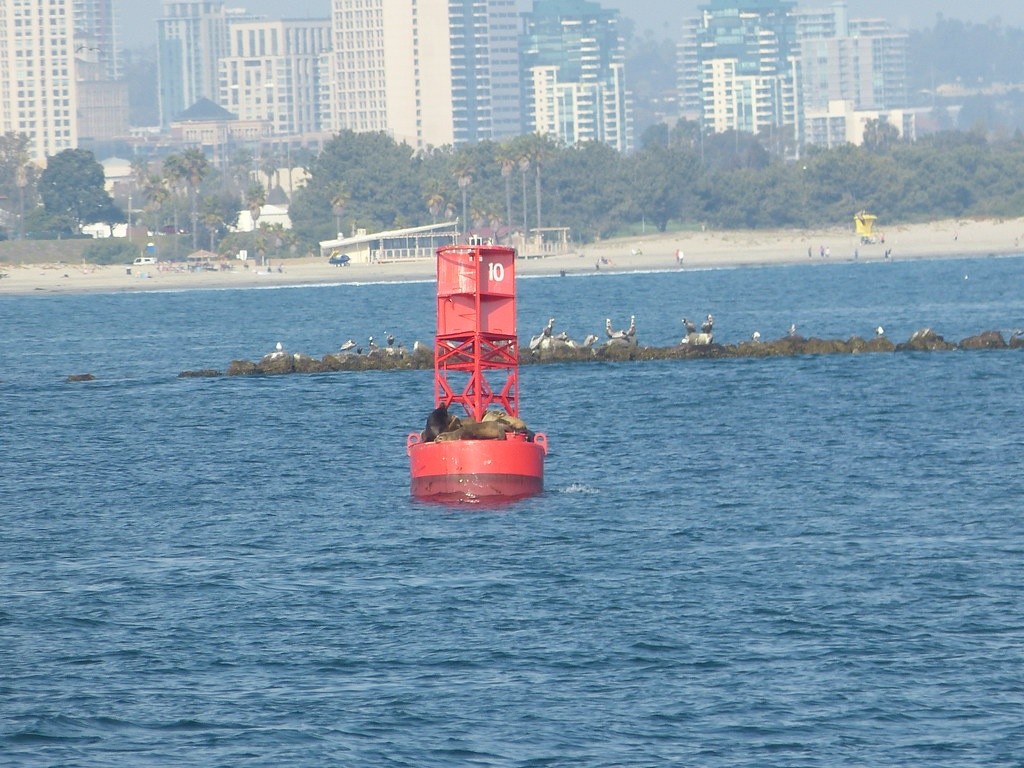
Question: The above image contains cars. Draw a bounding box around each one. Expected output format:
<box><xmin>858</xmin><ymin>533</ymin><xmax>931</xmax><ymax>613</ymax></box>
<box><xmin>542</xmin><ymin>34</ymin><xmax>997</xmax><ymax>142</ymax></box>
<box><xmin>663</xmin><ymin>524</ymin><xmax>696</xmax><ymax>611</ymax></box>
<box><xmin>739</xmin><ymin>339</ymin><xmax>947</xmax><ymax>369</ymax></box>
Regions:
<box><xmin>133</xmin><ymin>257</ymin><xmax>155</xmax><ymax>266</ymax></box>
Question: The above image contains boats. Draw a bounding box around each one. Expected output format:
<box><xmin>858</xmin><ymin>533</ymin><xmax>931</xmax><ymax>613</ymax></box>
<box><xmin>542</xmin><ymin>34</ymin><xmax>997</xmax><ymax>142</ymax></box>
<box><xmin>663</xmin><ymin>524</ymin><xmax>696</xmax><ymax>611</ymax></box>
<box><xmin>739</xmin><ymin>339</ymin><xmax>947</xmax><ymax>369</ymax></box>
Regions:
<box><xmin>408</xmin><ymin>245</ymin><xmax>546</xmax><ymax>496</ymax></box>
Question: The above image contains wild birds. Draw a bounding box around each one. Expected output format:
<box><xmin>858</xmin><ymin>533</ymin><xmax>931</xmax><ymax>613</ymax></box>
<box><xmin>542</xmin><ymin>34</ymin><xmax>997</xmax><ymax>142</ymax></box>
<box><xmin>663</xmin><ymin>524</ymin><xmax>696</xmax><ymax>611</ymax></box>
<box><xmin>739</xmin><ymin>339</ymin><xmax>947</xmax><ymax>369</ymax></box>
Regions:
<box><xmin>750</xmin><ymin>330</ymin><xmax>760</xmax><ymax>343</ymax></box>
<box><xmin>787</xmin><ymin>323</ymin><xmax>796</xmax><ymax>335</ymax></box>
<box><xmin>542</xmin><ymin>314</ymin><xmax>636</xmax><ymax>339</ymax></box>
<box><xmin>875</xmin><ymin>326</ymin><xmax>883</xmax><ymax>336</ymax></box>
<box><xmin>681</xmin><ymin>314</ymin><xmax>713</xmax><ymax>334</ymax></box>
<box><xmin>275</xmin><ymin>341</ymin><xmax>283</xmax><ymax>351</ymax></box>
<box><xmin>338</xmin><ymin>334</ymin><xmax>419</xmax><ymax>354</ymax></box>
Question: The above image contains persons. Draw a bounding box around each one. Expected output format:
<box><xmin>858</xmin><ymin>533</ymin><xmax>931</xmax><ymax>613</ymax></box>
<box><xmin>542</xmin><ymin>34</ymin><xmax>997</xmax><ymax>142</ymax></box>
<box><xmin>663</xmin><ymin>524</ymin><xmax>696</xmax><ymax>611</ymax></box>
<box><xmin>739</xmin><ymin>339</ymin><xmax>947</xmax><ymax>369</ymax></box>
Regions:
<box><xmin>954</xmin><ymin>232</ymin><xmax>958</xmax><ymax>239</ymax></box>
<box><xmin>1015</xmin><ymin>238</ymin><xmax>1020</xmax><ymax>247</ymax></box>
<box><xmin>701</xmin><ymin>315</ymin><xmax>714</xmax><ymax>344</ymax></box>
<box><xmin>682</xmin><ymin>318</ymin><xmax>696</xmax><ymax>333</ymax></box>
<box><xmin>676</xmin><ymin>249</ymin><xmax>684</xmax><ymax>265</ymax></box>
<box><xmin>809</xmin><ymin>233</ymin><xmax>892</xmax><ymax>263</ymax></box>
<box><xmin>425</xmin><ymin>406</ymin><xmax>448</xmax><ymax>442</ymax></box>
